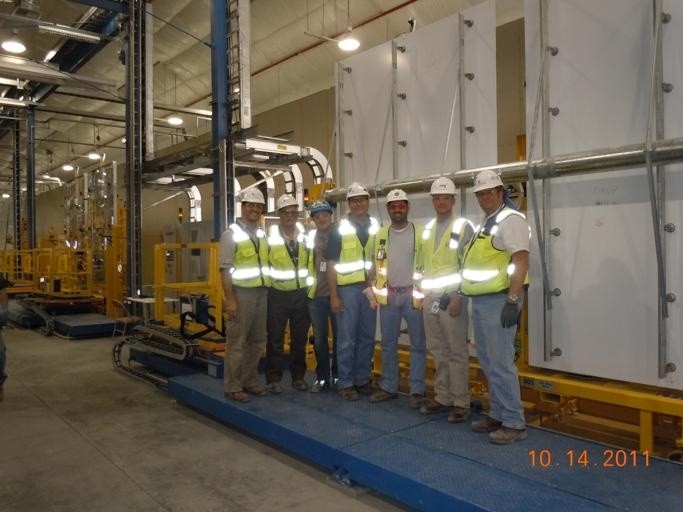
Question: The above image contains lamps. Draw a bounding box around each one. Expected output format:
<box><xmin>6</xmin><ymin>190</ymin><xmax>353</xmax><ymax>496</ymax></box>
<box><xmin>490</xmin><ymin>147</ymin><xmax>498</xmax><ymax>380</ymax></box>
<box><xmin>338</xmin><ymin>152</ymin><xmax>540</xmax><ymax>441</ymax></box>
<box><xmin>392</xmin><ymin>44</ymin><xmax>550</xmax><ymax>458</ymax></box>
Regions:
<box><xmin>0</xmin><ymin>0</ymin><xmax>184</xmax><ymax>178</ymax></box>
<box><xmin>338</xmin><ymin>0</ymin><xmax>360</xmax><ymax>51</ymax></box>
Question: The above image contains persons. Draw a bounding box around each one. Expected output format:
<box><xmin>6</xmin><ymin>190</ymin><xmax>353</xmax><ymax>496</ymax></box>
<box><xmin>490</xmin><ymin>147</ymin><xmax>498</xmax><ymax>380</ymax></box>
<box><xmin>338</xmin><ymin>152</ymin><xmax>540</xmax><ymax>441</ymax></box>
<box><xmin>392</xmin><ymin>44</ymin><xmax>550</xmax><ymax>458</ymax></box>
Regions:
<box><xmin>0</xmin><ymin>273</ymin><xmax>9</xmax><ymax>405</ymax></box>
<box><xmin>266</xmin><ymin>194</ymin><xmax>312</xmax><ymax>395</ymax></box>
<box><xmin>364</xmin><ymin>188</ymin><xmax>425</xmax><ymax>410</ymax></box>
<box><xmin>414</xmin><ymin>176</ymin><xmax>477</xmax><ymax>425</ymax></box>
<box><xmin>460</xmin><ymin>168</ymin><xmax>531</xmax><ymax>445</ymax></box>
<box><xmin>326</xmin><ymin>184</ymin><xmax>381</xmax><ymax>401</ymax></box>
<box><xmin>218</xmin><ymin>186</ymin><xmax>270</xmax><ymax>403</ymax></box>
<box><xmin>305</xmin><ymin>198</ymin><xmax>338</xmax><ymax>396</ymax></box>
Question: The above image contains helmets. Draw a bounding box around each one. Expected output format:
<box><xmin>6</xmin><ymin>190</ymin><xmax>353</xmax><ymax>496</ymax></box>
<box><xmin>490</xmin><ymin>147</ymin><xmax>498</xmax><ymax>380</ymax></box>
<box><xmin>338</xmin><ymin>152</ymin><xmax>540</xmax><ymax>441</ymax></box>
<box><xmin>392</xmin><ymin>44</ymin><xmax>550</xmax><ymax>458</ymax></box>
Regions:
<box><xmin>429</xmin><ymin>177</ymin><xmax>457</xmax><ymax>195</ymax></box>
<box><xmin>472</xmin><ymin>170</ymin><xmax>505</xmax><ymax>194</ymax></box>
<box><xmin>345</xmin><ymin>182</ymin><xmax>409</xmax><ymax>205</ymax></box>
<box><xmin>241</xmin><ymin>187</ymin><xmax>332</xmax><ymax>217</ymax></box>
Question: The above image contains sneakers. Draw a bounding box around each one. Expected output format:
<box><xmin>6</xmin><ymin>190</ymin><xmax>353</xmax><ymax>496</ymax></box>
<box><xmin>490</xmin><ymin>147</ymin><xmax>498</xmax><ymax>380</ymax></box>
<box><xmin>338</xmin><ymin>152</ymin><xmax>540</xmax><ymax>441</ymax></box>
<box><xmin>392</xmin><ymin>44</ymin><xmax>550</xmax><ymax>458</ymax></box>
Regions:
<box><xmin>226</xmin><ymin>377</ymin><xmax>471</xmax><ymax>422</ymax></box>
<box><xmin>489</xmin><ymin>426</ymin><xmax>528</xmax><ymax>444</ymax></box>
<box><xmin>471</xmin><ymin>419</ymin><xmax>502</xmax><ymax>432</ymax></box>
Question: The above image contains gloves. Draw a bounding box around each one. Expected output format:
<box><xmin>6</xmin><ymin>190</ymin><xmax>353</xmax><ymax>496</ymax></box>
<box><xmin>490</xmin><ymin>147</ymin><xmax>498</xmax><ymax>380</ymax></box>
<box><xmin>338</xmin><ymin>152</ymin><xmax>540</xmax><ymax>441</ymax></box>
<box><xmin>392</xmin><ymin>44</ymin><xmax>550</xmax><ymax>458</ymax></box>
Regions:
<box><xmin>501</xmin><ymin>303</ymin><xmax>518</xmax><ymax>329</ymax></box>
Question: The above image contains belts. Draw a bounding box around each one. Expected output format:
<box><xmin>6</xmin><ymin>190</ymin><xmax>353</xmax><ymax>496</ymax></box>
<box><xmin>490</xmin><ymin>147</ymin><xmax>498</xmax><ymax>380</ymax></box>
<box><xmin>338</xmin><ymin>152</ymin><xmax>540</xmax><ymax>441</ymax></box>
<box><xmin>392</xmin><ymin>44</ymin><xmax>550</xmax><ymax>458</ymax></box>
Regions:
<box><xmin>388</xmin><ymin>287</ymin><xmax>412</xmax><ymax>292</ymax></box>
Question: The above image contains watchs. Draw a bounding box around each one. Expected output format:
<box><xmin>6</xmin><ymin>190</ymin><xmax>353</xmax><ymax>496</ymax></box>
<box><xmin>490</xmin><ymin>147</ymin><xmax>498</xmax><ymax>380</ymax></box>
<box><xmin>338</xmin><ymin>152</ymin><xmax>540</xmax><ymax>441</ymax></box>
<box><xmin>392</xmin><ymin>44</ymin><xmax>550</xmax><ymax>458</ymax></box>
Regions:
<box><xmin>507</xmin><ymin>294</ymin><xmax>520</xmax><ymax>303</ymax></box>
